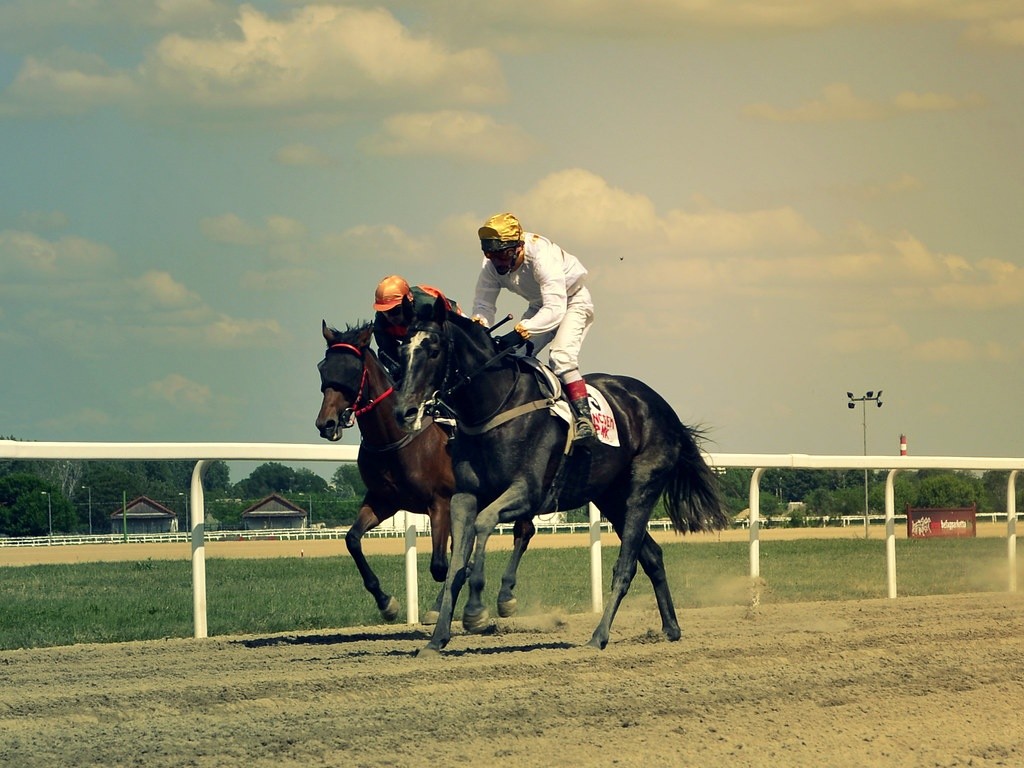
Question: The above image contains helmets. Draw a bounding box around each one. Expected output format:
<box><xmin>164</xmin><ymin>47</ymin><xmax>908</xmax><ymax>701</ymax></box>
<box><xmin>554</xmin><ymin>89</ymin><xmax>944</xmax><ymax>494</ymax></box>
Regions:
<box><xmin>373</xmin><ymin>275</ymin><xmax>413</xmax><ymax>311</ymax></box>
<box><xmin>478</xmin><ymin>213</ymin><xmax>525</xmax><ymax>251</ymax></box>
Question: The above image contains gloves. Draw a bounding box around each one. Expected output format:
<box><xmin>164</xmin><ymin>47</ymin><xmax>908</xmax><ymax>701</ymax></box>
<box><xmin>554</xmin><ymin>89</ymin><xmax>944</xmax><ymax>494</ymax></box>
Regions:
<box><xmin>499</xmin><ymin>330</ymin><xmax>524</xmax><ymax>355</ymax></box>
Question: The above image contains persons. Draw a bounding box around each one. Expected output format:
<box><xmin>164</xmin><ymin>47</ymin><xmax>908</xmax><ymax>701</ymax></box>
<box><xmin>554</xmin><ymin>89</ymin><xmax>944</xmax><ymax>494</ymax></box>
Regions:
<box><xmin>373</xmin><ymin>275</ymin><xmax>470</xmax><ymax>367</ymax></box>
<box><xmin>471</xmin><ymin>213</ymin><xmax>598</xmax><ymax>447</ymax></box>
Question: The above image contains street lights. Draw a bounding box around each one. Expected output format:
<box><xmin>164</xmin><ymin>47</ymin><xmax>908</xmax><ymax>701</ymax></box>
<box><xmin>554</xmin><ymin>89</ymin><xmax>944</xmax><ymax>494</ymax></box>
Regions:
<box><xmin>41</xmin><ymin>491</ymin><xmax>52</xmax><ymax>535</ymax></box>
<box><xmin>845</xmin><ymin>389</ymin><xmax>885</xmax><ymax>540</ymax></box>
<box><xmin>179</xmin><ymin>493</ymin><xmax>188</xmax><ymax>542</ymax></box>
<box><xmin>299</xmin><ymin>493</ymin><xmax>312</xmax><ymax>524</ymax></box>
<box><xmin>82</xmin><ymin>485</ymin><xmax>92</xmax><ymax>535</ymax></box>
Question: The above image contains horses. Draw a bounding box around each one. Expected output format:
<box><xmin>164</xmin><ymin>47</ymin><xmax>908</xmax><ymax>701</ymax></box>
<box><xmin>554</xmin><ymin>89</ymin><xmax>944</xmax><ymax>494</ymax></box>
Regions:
<box><xmin>311</xmin><ymin>293</ymin><xmax>748</xmax><ymax>665</ymax></box>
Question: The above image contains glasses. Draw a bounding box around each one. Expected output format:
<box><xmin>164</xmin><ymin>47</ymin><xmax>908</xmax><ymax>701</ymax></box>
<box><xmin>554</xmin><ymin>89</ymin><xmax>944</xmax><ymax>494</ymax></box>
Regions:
<box><xmin>484</xmin><ymin>248</ymin><xmax>514</xmax><ymax>260</ymax></box>
<box><xmin>383</xmin><ymin>306</ymin><xmax>402</xmax><ymax>318</ymax></box>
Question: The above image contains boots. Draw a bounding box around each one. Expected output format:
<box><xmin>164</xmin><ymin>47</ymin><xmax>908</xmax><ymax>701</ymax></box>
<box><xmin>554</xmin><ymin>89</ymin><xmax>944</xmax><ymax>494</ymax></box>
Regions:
<box><xmin>563</xmin><ymin>379</ymin><xmax>593</xmax><ymax>436</ymax></box>
